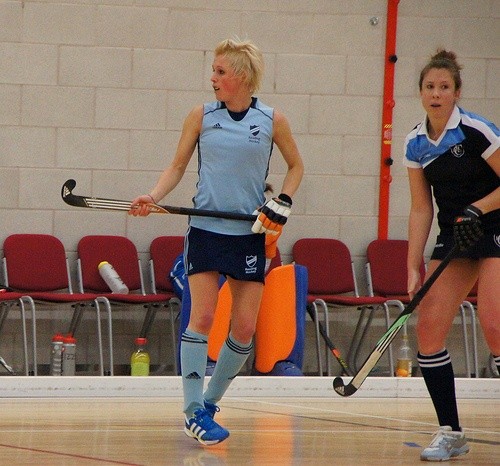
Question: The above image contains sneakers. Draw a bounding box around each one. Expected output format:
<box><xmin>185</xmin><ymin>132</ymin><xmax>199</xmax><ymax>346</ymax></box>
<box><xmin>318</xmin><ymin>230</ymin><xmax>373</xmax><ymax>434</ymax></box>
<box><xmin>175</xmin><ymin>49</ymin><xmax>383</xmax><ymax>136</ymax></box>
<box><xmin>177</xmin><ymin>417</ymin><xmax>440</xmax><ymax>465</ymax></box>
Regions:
<box><xmin>194</xmin><ymin>399</ymin><xmax>220</xmax><ymax>441</ymax></box>
<box><xmin>183</xmin><ymin>408</ymin><xmax>230</xmax><ymax>446</ymax></box>
<box><xmin>420</xmin><ymin>425</ymin><xmax>471</xmax><ymax>462</ymax></box>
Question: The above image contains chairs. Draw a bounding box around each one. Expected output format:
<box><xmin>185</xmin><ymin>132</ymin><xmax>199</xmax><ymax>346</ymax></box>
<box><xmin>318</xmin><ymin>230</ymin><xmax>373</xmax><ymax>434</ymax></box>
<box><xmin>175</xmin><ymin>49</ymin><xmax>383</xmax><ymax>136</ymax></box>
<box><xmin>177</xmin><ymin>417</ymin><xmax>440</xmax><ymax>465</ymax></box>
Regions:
<box><xmin>73</xmin><ymin>236</ymin><xmax>179</xmax><ymax>376</ymax></box>
<box><xmin>144</xmin><ymin>235</ymin><xmax>185</xmax><ymax>334</ymax></box>
<box><xmin>291</xmin><ymin>238</ymin><xmax>395</xmax><ymax>376</ymax></box>
<box><xmin>264</xmin><ymin>247</ymin><xmax>324</xmax><ymax>376</ymax></box>
<box><xmin>351</xmin><ymin>238</ymin><xmax>470</xmax><ymax>377</ymax></box>
<box><xmin>0</xmin><ymin>233</ymin><xmax>106</xmax><ymax>376</ymax></box>
<box><xmin>462</xmin><ymin>280</ymin><xmax>480</xmax><ymax>378</ymax></box>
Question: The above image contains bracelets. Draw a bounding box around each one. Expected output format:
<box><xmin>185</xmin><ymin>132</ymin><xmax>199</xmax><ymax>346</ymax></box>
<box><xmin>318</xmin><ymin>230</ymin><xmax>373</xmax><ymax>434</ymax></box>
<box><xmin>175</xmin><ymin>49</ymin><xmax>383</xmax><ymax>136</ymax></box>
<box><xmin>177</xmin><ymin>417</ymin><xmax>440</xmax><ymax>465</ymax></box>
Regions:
<box><xmin>148</xmin><ymin>195</ymin><xmax>156</xmax><ymax>204</ymax></box>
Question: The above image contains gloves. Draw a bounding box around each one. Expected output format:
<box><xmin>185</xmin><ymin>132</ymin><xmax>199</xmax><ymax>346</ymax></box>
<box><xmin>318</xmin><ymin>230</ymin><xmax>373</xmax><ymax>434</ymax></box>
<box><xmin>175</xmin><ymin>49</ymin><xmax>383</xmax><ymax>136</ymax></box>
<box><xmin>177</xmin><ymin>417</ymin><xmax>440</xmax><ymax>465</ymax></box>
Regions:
<box><xmin>251</xmin><ymin>194</ymin><xmax>293</xmax><ymax>236</ymax></box>
<box><xmin>453</xmin><ymin>205</ymin><xmax>484</xmax><ymax>253</ymax></box>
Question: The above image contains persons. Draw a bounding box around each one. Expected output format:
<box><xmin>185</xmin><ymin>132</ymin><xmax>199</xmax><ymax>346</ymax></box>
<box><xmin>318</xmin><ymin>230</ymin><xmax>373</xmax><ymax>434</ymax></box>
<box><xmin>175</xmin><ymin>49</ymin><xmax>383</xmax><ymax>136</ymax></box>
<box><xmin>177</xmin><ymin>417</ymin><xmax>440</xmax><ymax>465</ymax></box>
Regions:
<box><xmin>168</xmin><ymin>183</ymin><xmax>309</xmax><ymax>375</ymax></box>
<box><xmin>403</xmin><ymin>48</ymin><xmax>500</xmax><ymax>461</ymax></box>
<box><xmin>128</xmin><ymin>40</ymin><xmax>303</xmax><ymax>447</ymax></box>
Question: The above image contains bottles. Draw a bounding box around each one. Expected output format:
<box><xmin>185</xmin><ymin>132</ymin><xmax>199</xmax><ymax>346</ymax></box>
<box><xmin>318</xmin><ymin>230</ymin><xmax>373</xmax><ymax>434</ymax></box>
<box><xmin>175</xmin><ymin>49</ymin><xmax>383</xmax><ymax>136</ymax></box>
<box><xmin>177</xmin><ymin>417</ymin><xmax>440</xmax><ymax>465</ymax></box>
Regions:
<box><xmin>50</xmin><ymin>332</ymin><xmax>77</xmax><ymax>376</ymax></box>
<box><xmin>130</xmin><ymin>338</ymin><xmax>150</xmax><ymax>376</ymax></box>
<box><xmin>393</xmin><ymin>334</ymin><xmax>414</xmax><ymax>378</ymax></box>
<box><xmin>97</xmin><ymin>261</ymin><xmax>129</xmax><ymax>295</ymax></box>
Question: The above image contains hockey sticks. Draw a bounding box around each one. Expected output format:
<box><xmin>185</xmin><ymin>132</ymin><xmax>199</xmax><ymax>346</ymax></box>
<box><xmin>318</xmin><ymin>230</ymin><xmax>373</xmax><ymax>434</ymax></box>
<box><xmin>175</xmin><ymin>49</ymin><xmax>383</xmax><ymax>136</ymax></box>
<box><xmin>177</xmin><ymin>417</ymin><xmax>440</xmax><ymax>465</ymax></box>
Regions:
<box><xmin>61</xmin><ymin>178</ymin><xmax>258</xmax><ymax>220</ymax></box>
<box><xmin>332</xmin><ymin>242</ymin><xmax>461</xmax><ymax>398</ymax></box>
<box><xmin>306</xmin><ymin>304</ymin><xmax>356</xmax><ymax>376</ymax></box>
